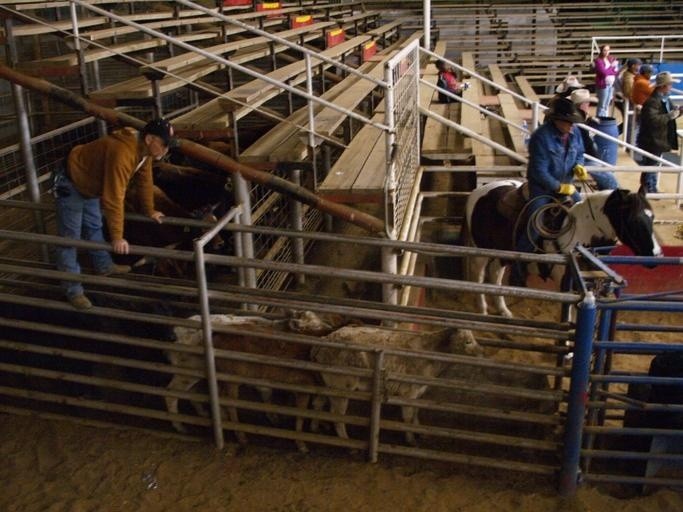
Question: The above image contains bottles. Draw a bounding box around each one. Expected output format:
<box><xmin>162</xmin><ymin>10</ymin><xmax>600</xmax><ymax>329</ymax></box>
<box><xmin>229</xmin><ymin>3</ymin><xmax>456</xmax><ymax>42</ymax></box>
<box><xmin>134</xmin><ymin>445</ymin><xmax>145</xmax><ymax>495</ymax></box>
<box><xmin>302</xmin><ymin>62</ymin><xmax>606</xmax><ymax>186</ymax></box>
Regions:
<box><xmin>519</xmin><ymin>119</ymin><xmax>530</xmax><ymax>156</ymax></box>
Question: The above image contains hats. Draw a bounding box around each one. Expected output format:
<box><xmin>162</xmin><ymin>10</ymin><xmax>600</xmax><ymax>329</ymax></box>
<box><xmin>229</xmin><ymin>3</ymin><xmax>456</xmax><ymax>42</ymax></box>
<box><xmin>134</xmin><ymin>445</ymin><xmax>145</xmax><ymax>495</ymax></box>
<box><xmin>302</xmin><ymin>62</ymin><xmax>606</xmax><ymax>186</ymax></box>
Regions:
<box><xmin>555</xmin><ymin>76</ymin><xmax>586</xmax><ymax>93</ymax></box>
<box><xmin>648</xmin><ymin>71</ymin><xmax>680</xmax><ymax>89</ymax></box>
<box><xmin>143</xmin><ymin>117</ymin><xmax>176</xmax><ymax>145</ymax></box>
<box><xmin>542</xmin><ymin>96</ymin><xmax>587</xmax><ymax>123</ymax></box>
<box><xmin>565</xmin><ymin>88</ymin><xmax>600</xmax><ymax>104</ymax></box>
<box><xmin>628</xmin><ymin>58</ymin><xmax>653</xmax><ymax>73</ymax></box>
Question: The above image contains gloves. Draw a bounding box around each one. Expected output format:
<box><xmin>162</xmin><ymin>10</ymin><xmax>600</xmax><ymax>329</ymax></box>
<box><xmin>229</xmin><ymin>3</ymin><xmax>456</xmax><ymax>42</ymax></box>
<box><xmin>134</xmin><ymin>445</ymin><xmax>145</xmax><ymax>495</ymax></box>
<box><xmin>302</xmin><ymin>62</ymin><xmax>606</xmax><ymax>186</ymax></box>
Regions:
<box><xmin>573</xmin><ymin>164</ymin><xmax>588</xmax><ymax>182</ymax></box>
<box><xmin>557</xmin><ymin>183</ymin><xmax>577</xmax><ymax>197</ymax></box>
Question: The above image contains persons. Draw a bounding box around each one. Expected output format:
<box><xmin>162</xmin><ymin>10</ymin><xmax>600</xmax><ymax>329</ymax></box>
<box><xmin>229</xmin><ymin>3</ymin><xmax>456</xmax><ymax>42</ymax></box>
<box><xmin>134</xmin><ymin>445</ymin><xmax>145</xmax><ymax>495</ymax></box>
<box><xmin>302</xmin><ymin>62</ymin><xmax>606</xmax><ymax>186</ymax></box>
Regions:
<box><xmin>51</xmin><ymin>117</ymin><xmax>173</xmax><ymax>308</ymax></box>
<box><xmin>637</xmin><ymin>72</ymin><xmax>683</xmax><ymax>200</ymax></box>
<box><xmin>435</xmin><ymin>60</ymin><xmax>470</xmax><ymax>102</ymax></box>
<box><xmin>507</xmin><ymin>96</ymin><xmax>585</xmax><ymax>286</ymax></box>
<box><xmin>595</xmin><ymin>45</ymin><xmax>620</xmax><ymax>118</ymax></box>
<box><xmin>629</xmin><ymin>64</ymin><xmax>655</xmax><ymax>163</ymax></box>
<box><xmin>570</xmin><ymin>88</ymin><xmax>600</xmax><ymax>158</ymax></box>
<box><xmin>613</xmin><ymin>59</ymin><xmax>628</xmax><ymax>135</ymax></box>
<box><xmin>623</xmin><ymin>57</ymin><xmax>642</xmax><ymax>151</ymax></box>
<box><xmin>547</xmin><ymin>76</ymin><xmax>585</xmax><ymax>107</ymax></box>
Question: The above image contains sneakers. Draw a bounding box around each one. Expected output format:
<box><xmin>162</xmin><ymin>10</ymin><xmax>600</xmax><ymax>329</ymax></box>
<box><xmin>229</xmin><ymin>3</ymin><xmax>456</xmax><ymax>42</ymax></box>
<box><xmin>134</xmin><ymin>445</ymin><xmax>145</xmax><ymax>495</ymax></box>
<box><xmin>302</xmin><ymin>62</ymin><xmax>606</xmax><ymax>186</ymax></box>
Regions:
<box><xmin>63</xmin><ymin>290</ymin><xmax>92</xmax><ymax>309</ymax></box>
<box><xmin>103</xmin><ymin>263</ymin><xmax>132</xmax><ymax>276</ymax></box>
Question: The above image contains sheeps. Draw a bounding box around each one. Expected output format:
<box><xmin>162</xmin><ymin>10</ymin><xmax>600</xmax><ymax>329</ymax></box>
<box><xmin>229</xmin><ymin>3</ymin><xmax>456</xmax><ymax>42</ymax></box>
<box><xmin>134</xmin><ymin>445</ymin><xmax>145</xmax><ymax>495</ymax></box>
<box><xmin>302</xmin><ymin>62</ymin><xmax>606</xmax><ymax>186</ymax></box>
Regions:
<box><xmin>165</xmin><ymin>310</ymin><xmax>366</xmax><ymax>454</ymax></box>
<box><xmin>313</xmin><ymin>325</ymin><xmax>483</xmax><ymax>442</ymax></box>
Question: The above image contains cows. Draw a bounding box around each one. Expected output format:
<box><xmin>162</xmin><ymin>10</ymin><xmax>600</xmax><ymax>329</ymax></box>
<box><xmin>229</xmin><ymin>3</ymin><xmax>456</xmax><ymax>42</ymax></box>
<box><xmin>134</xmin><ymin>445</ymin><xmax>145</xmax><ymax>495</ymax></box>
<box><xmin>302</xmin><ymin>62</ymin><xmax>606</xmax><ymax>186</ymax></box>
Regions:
<box><xmin>154</xmin><ymin>139</ymin><xmax>243</xmax><ymax>198</ymax></box>
<box><xmin>464</xmin><ymin>180</ymin><xmax>664</xmax><ymax>320</ymax></box>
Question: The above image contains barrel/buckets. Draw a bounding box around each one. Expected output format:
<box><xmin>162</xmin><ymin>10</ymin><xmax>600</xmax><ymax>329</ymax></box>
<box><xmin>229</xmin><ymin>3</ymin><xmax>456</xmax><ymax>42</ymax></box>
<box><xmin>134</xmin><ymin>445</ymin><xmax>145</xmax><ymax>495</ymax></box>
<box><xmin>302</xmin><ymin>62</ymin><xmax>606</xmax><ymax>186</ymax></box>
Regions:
<box><xmin>585</xmin><ymin>116</ymin><xmax>619</xmax><ymax>166</ymax></box>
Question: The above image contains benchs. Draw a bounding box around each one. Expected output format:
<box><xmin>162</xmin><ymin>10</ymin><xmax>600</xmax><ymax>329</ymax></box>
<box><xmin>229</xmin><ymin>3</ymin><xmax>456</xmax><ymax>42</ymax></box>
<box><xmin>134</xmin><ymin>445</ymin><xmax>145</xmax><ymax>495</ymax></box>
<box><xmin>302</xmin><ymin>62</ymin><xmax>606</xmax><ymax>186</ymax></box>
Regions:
<box><xmin>516</xmin><ymin>56</ymin><xmax>596</xmax><ymax>98</ymax></box>
<box><xmin>2</xmin><ymin>2</ymin><xmax>447</xmax><ymax>207</ymax></box>
<box><xmin>459</xmin><ymin>3</ymin><xmax>545</xmax><ymax>205</ymax></box>
<box><xmin>489</xmin><ymin>5</ymin><xmax>683</xmax><ymax>60</ymax></box>
